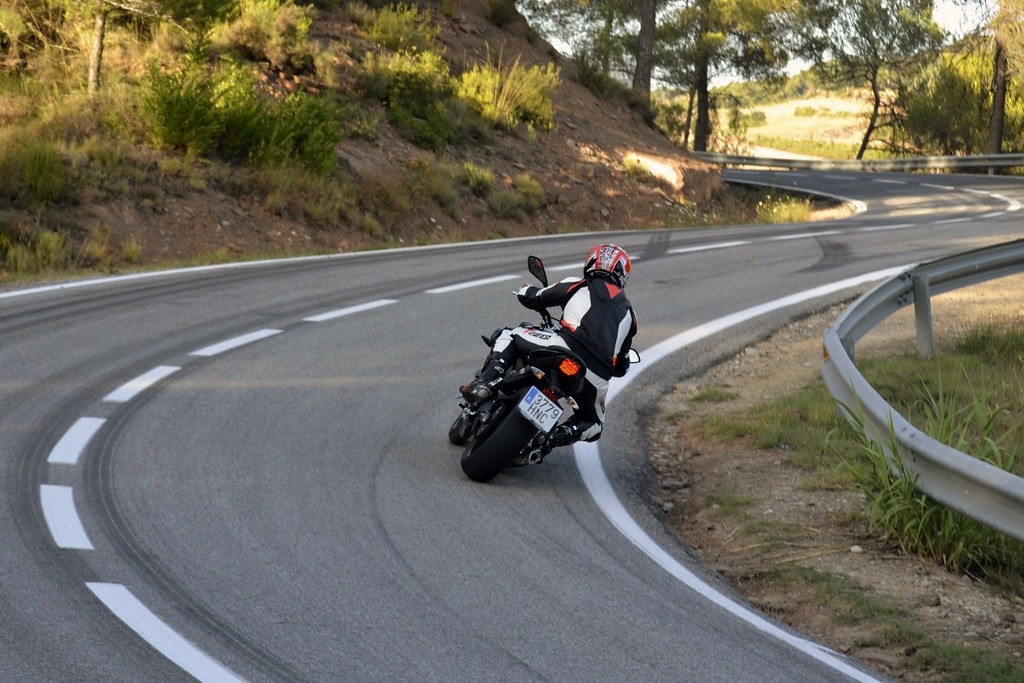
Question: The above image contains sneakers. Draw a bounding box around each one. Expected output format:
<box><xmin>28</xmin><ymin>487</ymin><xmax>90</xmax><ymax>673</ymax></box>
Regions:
<box><xmin>459</xmin><ymin>382</ymin><xmax>492</xmax><ymax>405</ymax></box>
<box><xmin>560</xmin><ymin>423</ymin><xmax>578</xmax><ymax>449</ymax></box>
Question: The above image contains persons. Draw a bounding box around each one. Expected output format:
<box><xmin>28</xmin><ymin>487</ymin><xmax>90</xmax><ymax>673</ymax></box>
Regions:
<box><xmin>459</xmin><ymin>243</ymin><xmax>637</xmax><ymax>465</ymax></box>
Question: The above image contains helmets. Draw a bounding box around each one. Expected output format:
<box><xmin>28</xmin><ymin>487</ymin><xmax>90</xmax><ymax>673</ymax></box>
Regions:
<box><xmin>583</xmin><ymin>243</ymin><xmax>631</xmax><ymax>289</ymax></box>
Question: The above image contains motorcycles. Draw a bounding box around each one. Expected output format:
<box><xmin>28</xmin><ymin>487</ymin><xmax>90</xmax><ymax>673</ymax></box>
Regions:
<box><xmin>448</xmin><ymin>255</ymin><xmax>642</xmax><ymax>483</ymax></box>
